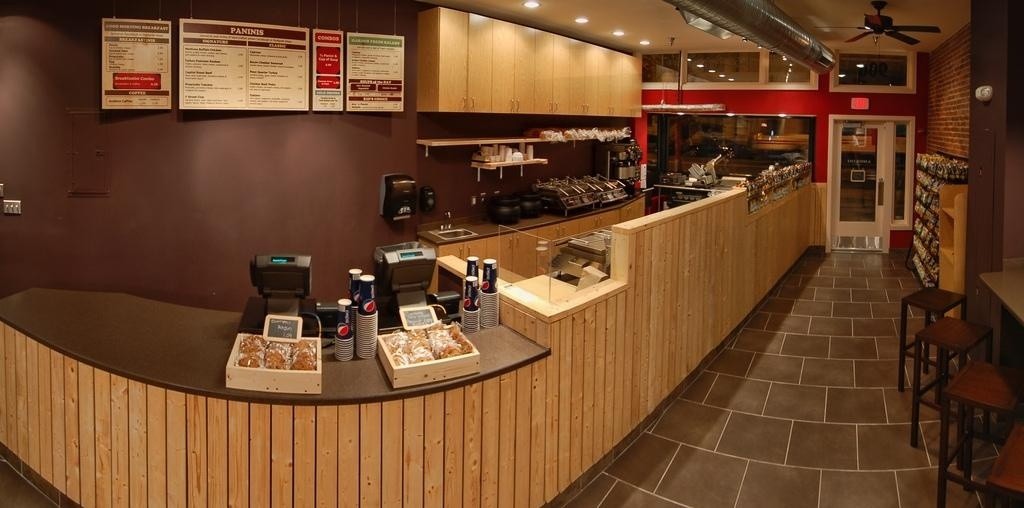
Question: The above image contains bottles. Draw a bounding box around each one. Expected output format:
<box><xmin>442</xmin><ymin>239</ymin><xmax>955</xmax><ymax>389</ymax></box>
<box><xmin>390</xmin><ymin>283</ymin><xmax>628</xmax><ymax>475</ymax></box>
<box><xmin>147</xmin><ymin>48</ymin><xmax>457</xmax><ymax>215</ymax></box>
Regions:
<box><xmin>739</xmin><ymin>161</ymin><xmax>812</xmax><ymax>212</ymax></box>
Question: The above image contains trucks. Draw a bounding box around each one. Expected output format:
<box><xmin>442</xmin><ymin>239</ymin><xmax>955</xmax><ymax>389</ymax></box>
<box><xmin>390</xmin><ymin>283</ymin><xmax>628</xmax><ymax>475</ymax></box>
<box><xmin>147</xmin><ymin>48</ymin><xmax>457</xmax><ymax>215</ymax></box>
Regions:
<box><xmin>749</xmin><ymin>127</ymin><xmax>907</xmax><ymax>180</ymax></box>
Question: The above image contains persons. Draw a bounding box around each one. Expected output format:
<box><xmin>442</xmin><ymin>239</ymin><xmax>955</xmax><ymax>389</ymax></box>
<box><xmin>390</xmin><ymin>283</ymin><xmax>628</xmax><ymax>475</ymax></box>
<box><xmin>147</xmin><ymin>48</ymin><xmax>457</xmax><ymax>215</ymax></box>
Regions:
<box><xmin>683</xmin><ymin>131</ymin><xmax>704</xmax><ymax>157</ymax></box>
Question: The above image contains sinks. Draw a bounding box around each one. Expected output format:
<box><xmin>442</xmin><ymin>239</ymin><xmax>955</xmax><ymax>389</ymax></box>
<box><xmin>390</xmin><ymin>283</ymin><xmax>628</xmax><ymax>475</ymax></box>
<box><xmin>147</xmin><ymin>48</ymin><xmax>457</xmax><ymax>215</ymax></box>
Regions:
<box><xmin>428</xmin><ymin>228</ymin><xmax>478</xmax><ymax>240</ymax></box>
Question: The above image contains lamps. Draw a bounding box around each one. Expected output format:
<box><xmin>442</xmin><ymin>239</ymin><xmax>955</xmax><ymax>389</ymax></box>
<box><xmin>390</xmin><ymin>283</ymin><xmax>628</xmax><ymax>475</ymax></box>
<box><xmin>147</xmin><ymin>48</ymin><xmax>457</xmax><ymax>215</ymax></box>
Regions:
<box><xmin>642</xmin><ymin>55</ymin><xmax>727</xmax><ymax>113</ymax></box>
<box><xmin>871</xmin><ymin>24</ymin><xmax>885</xmax><ymax>57</ymax></box>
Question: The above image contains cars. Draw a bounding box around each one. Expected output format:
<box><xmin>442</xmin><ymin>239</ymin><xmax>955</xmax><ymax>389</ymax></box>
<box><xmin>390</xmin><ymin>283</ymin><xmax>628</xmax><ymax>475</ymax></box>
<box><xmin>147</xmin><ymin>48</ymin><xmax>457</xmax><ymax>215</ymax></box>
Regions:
<box><xmin>649</xmin><ymin>134</ymin><xmax>752</xmax><ymax>160</ymax></box>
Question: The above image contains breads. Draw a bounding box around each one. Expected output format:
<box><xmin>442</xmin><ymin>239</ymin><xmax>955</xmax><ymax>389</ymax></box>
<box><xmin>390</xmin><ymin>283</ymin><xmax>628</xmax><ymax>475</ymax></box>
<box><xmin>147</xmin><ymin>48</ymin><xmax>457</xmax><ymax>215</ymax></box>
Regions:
<box><xmin>238</xmin><ymin>334</ymin><xmax>318</xmax><ymax>371</ymax></box>
<box><xmin>388</xmin><ymin>322</ymin><xmax>474</xmax><ymax>367</ymax></box>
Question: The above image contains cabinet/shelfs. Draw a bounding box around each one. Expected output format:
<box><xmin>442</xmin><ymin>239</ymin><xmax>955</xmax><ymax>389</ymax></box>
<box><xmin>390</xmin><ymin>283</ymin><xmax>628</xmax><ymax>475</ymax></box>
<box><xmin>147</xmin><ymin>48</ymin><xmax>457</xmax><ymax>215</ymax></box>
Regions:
<box><xmin>535</xmin><ymin>28</ymin><xmax>572</xmax><ymax>116</ymax></box>
<box><xmin>417</xmin><ymin>6</ymin><xmax>492</xmax><ymax>114</ymax></box>
<box><xmin>937</xmin><ymin>183</ymin><xmax>968</xmax><ymax>322</ymax></box>
<box><xmin>417</xmin><ymin>193</ymin><xmax>647</xmax><ymax>280</ymax></box>
<box><xmin>611</xmin><ymin>49</ymin><xmax>642</xmax><ymax>118</ymax></box>
<box><xmin>492</xmin><ymin>18</ymin><xmax>535</xmax><ymax>116</ymax></box>
<box><xmin>573</xmin><ymin>38</ymin><xmax>610</xmax><ymax>118</ymax></box>
<box><xmin>415</xmin><ymin>135</ymin><xmax>613</xmax><ymax>181</ymax></box>
<box><xmin>906</xmin><ymin>151</ymin><xmax>968</xmax><ymax>287</ymax></box>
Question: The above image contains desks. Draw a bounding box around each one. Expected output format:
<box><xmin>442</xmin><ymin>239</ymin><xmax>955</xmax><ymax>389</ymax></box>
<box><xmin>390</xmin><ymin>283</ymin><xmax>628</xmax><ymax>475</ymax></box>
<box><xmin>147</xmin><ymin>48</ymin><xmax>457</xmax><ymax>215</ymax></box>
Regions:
<box><xmin>976</xmin><ymin>270</ymin><xmax>1024</xmax><ymax>372</ymax></box>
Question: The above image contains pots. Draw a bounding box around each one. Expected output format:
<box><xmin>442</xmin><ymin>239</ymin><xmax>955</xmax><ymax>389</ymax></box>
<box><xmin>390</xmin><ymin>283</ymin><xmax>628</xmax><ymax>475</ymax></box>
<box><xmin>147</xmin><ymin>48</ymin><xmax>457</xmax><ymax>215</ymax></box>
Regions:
<box><xmin>487</xmin><ymin>190</ymin><xmax>542</xmax><ymax>223</ymax></box>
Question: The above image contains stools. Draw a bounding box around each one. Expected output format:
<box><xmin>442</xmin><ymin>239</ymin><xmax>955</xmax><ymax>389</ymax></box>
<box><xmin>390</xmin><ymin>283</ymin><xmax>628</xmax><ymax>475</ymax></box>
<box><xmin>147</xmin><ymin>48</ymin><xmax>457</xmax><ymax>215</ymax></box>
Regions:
<box><xmin>984</xmin><ymin>404</ymin><xmax>1024</xmax><ymax>508</ymax></box>
<box><xmin>898</xmin><ymin>286</ymin><xmax>968</xmax><ymax>393</ymax></box>
<box><xmin>937</xmin><ymin>356</ymin><xmax>1024</xmax><ymax>507</ymax></box>
<box><xmin>910</xmin><ymin>315</ymin><xmax>993</xmax><ymax>471</ymax></box>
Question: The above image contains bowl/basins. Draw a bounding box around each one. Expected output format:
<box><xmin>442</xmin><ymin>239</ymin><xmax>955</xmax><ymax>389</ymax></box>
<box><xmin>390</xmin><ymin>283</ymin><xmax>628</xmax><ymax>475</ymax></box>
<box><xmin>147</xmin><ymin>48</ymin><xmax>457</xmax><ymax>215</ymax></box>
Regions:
<box><xmin>675</xmin><ymin>191</ymin><xmax>707</xmax><ymax>201</ymax></box>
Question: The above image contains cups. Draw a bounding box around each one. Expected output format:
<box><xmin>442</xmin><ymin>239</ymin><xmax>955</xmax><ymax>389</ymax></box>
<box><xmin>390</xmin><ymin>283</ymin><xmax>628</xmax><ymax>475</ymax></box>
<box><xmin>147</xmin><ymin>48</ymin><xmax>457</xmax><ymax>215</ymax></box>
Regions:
<box><xmin>332</xmin><ymin>269</ymin><xmax>379</xmax><ymax>363</ymax></box>
<box><xmin>480</xmin><ymin>143</ymin><xmax>533</xmax><ymax>161</ymax></box>
<box><xmin>461</xmin><ymin>255</ymin><xmax>501</xmax><ymax>334</ymax></box>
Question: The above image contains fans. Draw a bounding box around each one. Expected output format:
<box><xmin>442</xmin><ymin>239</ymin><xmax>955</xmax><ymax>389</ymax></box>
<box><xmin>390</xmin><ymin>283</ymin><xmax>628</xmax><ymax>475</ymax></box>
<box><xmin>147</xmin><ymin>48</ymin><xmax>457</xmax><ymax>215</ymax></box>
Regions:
<box><xmin>810</xmin><ymin>0</ymin><xmax>941</xmax><ymax>58</ymax></box>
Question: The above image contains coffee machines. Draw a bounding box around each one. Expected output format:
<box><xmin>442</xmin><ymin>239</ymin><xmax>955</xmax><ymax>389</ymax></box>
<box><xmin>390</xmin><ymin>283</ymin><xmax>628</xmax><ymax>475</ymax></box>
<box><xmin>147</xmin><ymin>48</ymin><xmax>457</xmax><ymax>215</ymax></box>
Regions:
<box><xmin>595</xmin><ymin>137</ymin><xmax>642</xmax><ymax>198</ymax></box>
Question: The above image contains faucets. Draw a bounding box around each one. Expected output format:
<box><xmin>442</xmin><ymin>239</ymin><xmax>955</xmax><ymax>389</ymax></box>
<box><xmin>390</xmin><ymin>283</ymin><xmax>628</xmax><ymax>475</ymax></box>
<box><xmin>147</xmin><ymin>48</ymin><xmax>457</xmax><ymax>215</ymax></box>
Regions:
<box><xmin>444</xmin><ymin>211</ymin><xmax>451</xmax><ymax>230</ymax></box>
